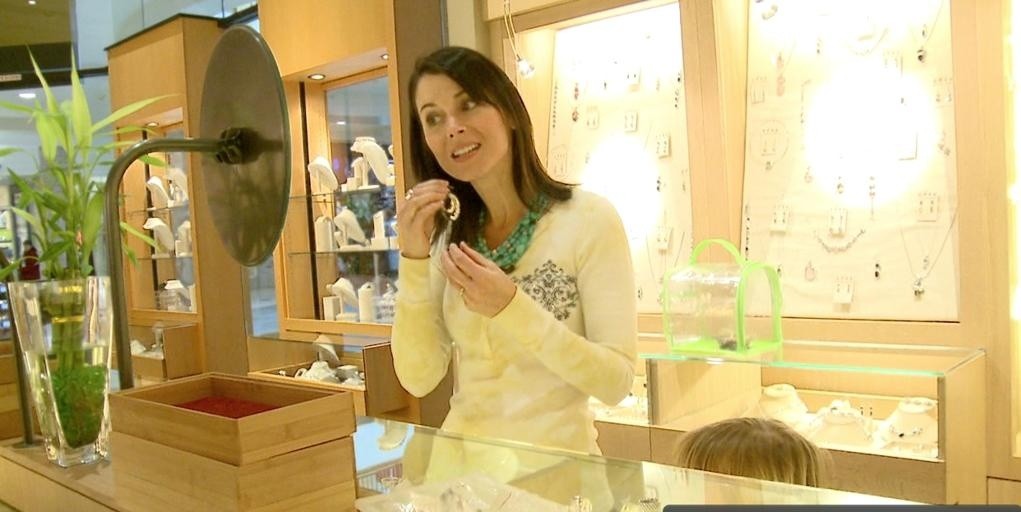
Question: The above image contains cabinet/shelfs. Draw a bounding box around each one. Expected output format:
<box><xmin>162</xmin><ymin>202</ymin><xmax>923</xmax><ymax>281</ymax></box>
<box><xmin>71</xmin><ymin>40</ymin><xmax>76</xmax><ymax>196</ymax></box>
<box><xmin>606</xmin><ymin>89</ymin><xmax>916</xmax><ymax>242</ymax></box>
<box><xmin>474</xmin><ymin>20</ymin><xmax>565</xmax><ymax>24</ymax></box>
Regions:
<box><xmin>586</xmin><ymin>332</ymin><xmax>990</xmax><ymax>509</ymax></box>
<box><xmin>103</xmin><ymin>12</ymin><xmax>249</xmax><ymax>382</ymax></box>
<box><xmin>0</xmin><ymin>390</ymin><xmax>926</xmax><ymax>510</ymax></box>
<box><xmin>253</xmin><ymin>1</ymin><xmax>453</xmax><ymax>429</ymax></box>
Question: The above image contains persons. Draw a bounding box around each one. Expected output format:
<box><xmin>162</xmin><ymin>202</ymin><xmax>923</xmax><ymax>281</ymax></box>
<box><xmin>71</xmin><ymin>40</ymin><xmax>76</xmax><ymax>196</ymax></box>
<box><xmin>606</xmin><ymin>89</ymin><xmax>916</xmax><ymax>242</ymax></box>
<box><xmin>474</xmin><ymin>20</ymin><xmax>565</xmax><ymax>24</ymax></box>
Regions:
<box><xmin>391</xmin><ymin>47</ymin><xmax>642</xmax><ymax>510</ymax></box>
<box><xmin>669</xmin><ymin>417</ymin><xmax>822</xmax><ymax>490</ymax></box>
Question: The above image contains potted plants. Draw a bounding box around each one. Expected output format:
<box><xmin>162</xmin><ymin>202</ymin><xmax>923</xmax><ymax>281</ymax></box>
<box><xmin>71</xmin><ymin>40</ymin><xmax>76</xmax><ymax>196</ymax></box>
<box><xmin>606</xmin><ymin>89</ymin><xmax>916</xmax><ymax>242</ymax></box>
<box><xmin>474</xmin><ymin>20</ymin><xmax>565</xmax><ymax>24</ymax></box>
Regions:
<box><xmin>0</xmin><ymin>42</ymin><xmax>181</xmax><ymax>468</ymax></box>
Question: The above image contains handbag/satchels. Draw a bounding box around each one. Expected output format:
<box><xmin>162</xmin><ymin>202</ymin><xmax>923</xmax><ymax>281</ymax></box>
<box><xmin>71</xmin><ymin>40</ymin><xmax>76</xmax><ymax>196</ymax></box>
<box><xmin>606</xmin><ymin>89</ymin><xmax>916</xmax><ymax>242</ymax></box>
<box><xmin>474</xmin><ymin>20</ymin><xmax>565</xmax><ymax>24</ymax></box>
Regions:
<box><xmin>660</xmin><ymin>237</ymin><xmax>784</xmax><ymax>362</ymax></box>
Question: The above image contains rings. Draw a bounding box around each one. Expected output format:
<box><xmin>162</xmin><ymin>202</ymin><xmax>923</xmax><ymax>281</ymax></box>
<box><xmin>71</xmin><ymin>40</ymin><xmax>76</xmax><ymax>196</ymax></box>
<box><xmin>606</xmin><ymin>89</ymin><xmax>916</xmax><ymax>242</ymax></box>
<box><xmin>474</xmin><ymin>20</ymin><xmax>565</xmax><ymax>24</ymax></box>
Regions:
<box><xmin>457</xmin><ymin>286</ymin><xmax>464</xmax><ymax>295</ymax></box>
<box><xmin>404</xmin><ymin>185</ymin><xmax>415</xmax><ymax>201</ymax></box>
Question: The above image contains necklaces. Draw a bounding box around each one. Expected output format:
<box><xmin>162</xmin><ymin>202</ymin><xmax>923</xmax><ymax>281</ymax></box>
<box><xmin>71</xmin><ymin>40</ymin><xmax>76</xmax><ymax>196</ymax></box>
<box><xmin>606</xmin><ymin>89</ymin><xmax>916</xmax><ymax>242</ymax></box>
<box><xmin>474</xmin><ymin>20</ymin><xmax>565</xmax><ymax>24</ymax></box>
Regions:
<box><xmin>567</xmin><ymin>62</ymin><xmax>682</xmax><ymax>267</ymax></box>
<box><xmin>754</xmin><ymin>0</ymin><xmax>957</xmax><ymax>311</ymax></box>
<box><xmin>890</xmin><ymin>423</ymin><xmax>924</xmax><ymax>439</ymax></box>
<box><xmin>472</xmin><ymin>193</ymin><xmax>547</xmax><ymax>268</ymax></box>
